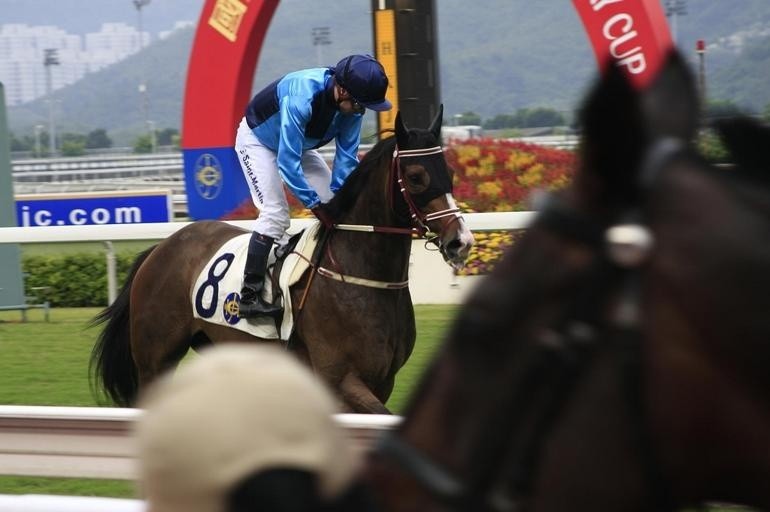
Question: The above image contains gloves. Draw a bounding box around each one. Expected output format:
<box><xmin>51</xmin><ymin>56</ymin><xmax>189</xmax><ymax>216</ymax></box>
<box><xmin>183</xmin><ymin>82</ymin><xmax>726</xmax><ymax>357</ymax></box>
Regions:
<box><xmin>313</xmin><ymin>201</ymin><xmax>335</xmax><ymax>231</ymax></box>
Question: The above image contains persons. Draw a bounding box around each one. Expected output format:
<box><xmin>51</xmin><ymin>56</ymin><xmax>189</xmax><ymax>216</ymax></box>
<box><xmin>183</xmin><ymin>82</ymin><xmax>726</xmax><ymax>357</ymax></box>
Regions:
<box><xmin>127</xmin><ymin>335</ymin><xmax>363</xmax><ymax>512</ymax></box>
<box><xmin>233</xmin><ymin>51</ymin><xmax>392</xmax><ymax>319</ymax></box>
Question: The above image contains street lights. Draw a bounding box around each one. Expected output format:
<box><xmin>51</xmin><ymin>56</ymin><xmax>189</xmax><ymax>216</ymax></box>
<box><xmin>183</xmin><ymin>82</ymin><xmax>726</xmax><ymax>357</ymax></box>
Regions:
<box><xmin>695</xmin><ymin>40</ymin><xmax>707</xmax><ymax>101</ymax></box>
<box><xmin>664</xmin><ymin>0</ymin><xmax>685</xmax><ymax>41</ymax></box>
<box><xmin>312</xmin><ymin>26</ymin><xmax>333</xmax><ymax>68</ymax></box>
<box><xmin>44</xmin><ymin>48</ymin><xmax>60</xmax><ymax>150</ymax></box>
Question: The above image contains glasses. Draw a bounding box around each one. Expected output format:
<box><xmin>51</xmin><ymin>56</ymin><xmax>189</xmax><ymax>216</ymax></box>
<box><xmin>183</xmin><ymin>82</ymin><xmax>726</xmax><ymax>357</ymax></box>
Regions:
<box><xmin>348</xmin><ymin>93</ymin><xmax>362</xmax><ymax>110</ymax></box>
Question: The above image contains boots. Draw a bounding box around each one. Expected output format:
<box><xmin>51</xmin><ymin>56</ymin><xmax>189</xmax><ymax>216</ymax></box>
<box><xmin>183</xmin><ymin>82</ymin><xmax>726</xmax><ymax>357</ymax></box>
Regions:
<box><xmin>240</xmin><ymin>232</ymin><xmax>282</xmax><ymax>319</ymax></box>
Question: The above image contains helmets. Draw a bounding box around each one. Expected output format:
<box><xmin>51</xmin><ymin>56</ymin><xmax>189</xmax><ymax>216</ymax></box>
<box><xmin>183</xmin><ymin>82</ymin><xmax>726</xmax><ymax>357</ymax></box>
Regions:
<box><xmin>337</xmin><ymin>54</ymin><xmax>393</xmax><ymax>112</ymax></box>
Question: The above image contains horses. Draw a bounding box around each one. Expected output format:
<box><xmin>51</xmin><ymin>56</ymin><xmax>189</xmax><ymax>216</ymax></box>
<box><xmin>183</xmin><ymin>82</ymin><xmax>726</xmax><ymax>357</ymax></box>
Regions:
<box><xmin>85</xmin><ymin>102</ymin><xmax>476</xmax><ymax>414</ymax></box>
<box><xmin>339</xmin><ymin>38</ymin><xmax>768</xmax><ymax>512</ymax></box>
